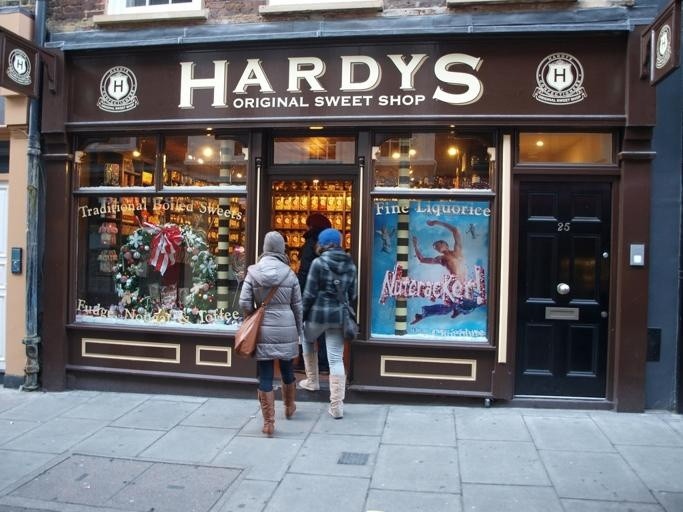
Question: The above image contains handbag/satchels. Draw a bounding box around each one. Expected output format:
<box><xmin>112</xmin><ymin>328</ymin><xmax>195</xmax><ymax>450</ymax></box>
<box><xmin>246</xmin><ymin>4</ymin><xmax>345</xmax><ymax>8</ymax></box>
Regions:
<box><xmin>234</xmin><ymin>307</ymin><xmax>265</xmax><ymax>358</ymax></box>
<box><xmin>341</xmin><ymin>306</ymin><xmax>360</xmax><ymax>341</ymax></box>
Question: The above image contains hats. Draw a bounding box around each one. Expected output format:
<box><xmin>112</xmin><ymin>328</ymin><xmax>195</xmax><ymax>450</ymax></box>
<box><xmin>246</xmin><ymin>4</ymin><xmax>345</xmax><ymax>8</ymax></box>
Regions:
<box><xmin>319</xmin><ymin>228</ymin><xmax>341</xmax><ymax>248</ymax></box>
<box><xmin>306</xmin><ymin>214</ymin><xmax>330</xmax><ymax>231</ymax></box>
<box><xmin>263</xmin><ymin>232</ymin><xmax>285</xmax><ymax>254</ymax></box>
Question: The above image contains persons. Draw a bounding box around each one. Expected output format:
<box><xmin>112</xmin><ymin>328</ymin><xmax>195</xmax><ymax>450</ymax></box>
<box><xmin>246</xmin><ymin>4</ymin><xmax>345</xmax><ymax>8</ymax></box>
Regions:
<box><xmin>296</xmin><ymin>226</ymin><xmax>356</xmax><ymax>422</ymax></box>
<box><xmin>294</xmin><ymin>212</ymin><xmax>331</xmax><ymax>376</ymax></box>
<box><xmin>239</xmin><ymin>230</ymin><xmax>304</xmax><ymax>435</ymax></box>
<box><xmin>408</xmin><ymin>220</ymin><xmax>477</xmax><ymax>326</ymax></box>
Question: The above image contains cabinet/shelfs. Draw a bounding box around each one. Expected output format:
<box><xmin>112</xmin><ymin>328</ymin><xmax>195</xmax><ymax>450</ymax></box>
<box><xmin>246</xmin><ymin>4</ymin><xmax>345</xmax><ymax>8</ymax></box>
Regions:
<box><xmin>87</xmin><ymin>153</ymin><xmax>353</xmax><ymax>276</ymax></box>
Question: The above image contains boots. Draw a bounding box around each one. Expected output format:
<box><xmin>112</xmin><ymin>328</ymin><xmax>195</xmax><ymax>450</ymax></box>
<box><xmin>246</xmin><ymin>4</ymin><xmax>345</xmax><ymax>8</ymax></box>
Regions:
<box><xmin>256</xmin><ymin>387</ymin><xmax>275</xmax><ymax>437</ymax></box>
<box><xmin>281</xmin><ymin>377</ymin><xmax>296</xmax><ymax>419</ymax></box>
<box><xmin>327</xmin><ymin>375</ymin><xmax>347</xmax><ymax>419</ymax></box>
<box><xmin>298</xmin><ymin>351</ymin><xmax>320</xmax><ymax>391</ymax></box>
